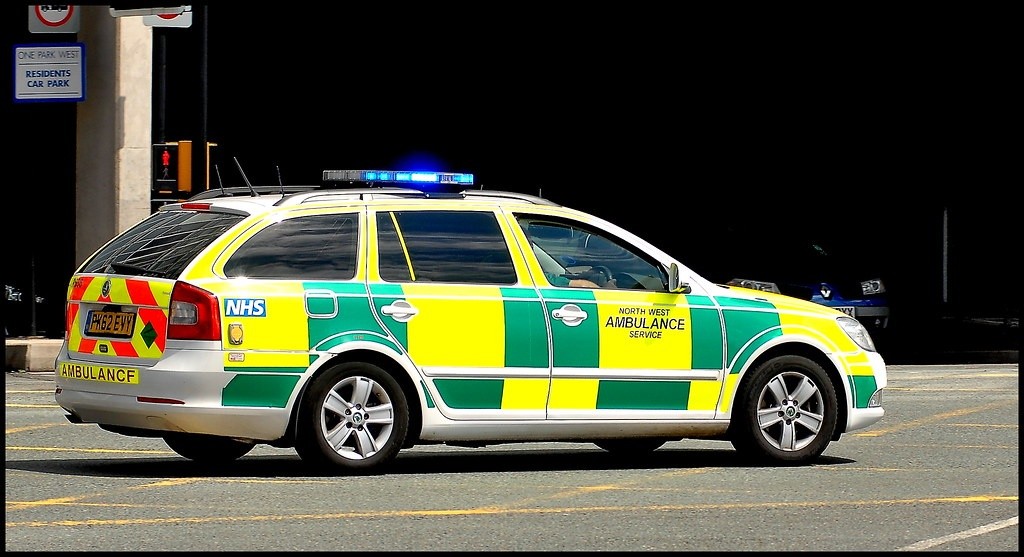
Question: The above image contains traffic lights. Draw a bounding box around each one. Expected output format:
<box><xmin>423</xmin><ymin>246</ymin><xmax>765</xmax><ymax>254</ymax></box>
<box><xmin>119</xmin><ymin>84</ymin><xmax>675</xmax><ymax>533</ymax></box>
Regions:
<box><xmin>152</xmin><ymin>140</ymin><xmax>193</xmax><ymax>193</ymax></box>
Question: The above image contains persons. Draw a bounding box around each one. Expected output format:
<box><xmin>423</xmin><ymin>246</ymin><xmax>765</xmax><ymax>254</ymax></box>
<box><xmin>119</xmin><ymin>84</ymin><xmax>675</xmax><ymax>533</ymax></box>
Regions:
<box><xmin>521</xmin><ymin>226</ymin><xmax>618</xmax><ymax>289</ymax></box>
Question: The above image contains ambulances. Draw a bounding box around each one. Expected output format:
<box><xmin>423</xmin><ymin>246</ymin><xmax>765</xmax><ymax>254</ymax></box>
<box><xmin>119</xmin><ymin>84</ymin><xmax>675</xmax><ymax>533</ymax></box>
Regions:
<box><xmin>56</xmin><ymin>169</ymin><xmax>888</xmax><ymax>476</ymax></box>
